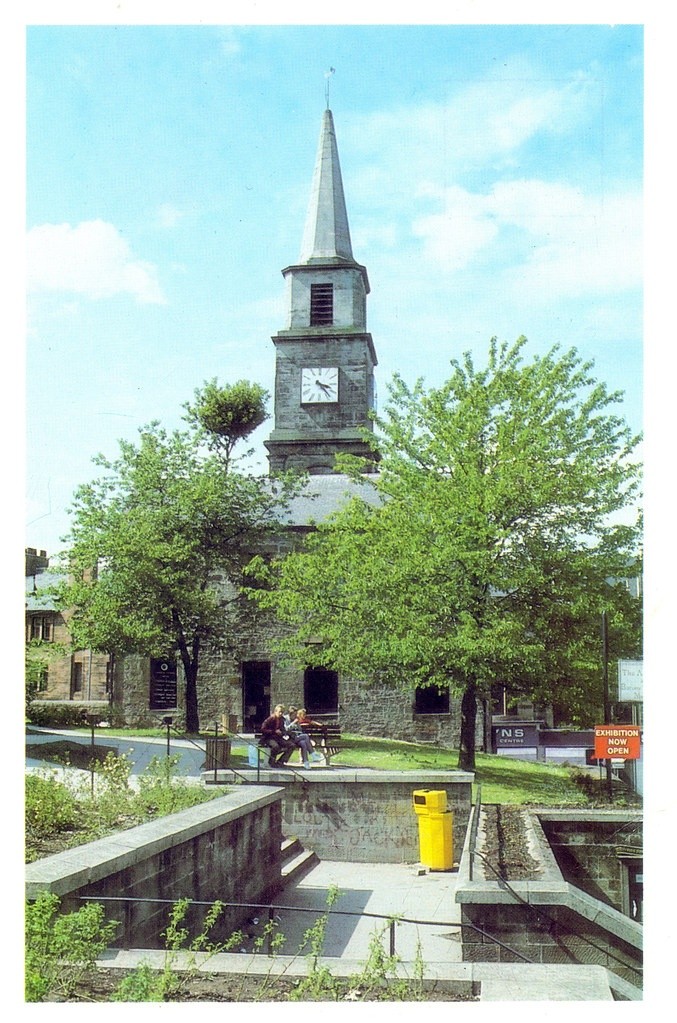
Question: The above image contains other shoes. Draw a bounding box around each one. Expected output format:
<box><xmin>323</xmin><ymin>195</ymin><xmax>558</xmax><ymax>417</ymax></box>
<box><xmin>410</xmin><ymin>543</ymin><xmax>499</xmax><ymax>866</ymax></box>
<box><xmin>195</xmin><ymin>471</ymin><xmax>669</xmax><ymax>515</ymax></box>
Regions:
<box><xmin>304</xmin><ymin>761</ymin><xmax>311</xmax><ymax>770</ymax></box>
<box><xmin>277</xmin><ymin>760</ymin><xmax>287</xmax><ymax>768</ymax></box>
<box><xmin>313</xmin><ymin>754</ymin><xmax>324</xmax><ymax>762</ymax></box>
<box><xmin>268</xmin><ymin>757</ymin><xmax>278</xmax><ymax>768</ymax></box>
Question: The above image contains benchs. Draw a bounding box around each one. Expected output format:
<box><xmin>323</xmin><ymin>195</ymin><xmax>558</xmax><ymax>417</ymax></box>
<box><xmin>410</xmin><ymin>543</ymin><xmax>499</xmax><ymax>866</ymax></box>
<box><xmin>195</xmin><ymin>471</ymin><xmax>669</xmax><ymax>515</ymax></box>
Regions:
<box><xmin>255</xmin><ymin>724</ymin><xmax>353</xmax><ymax>766</ymax></box>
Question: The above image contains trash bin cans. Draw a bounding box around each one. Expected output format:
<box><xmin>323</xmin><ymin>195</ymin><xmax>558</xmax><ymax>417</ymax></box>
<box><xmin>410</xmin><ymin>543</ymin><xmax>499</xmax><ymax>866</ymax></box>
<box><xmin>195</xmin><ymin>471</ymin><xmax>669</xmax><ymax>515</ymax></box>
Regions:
<box><xmin>413</xmin><ymin>788</ymin><xmax>453</xmax><ymax>870</ymax></box>
<box><xmin>206</xmin><ymin>739</ymin><xmax>231</xmax><ymax>770</ymax></box>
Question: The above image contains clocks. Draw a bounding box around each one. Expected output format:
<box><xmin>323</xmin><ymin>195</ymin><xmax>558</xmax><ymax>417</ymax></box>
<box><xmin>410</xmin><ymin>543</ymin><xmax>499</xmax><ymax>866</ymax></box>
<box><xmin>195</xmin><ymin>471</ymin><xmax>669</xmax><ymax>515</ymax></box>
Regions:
<box><xmin>300</xmin><ymin>367</ymin><xmax>339</xmax><ymax>403</ymax></box>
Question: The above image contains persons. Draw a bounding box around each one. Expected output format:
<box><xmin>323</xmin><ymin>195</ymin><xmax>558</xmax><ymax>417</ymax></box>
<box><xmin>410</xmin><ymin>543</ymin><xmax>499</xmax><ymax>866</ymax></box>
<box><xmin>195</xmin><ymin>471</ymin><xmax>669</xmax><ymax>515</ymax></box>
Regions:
<box><xmin>258</xmin><ymin>704</ymin><xmax>324</xmax><ymax>770</ymax></box>
